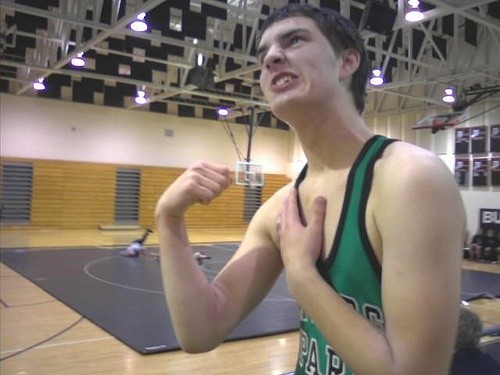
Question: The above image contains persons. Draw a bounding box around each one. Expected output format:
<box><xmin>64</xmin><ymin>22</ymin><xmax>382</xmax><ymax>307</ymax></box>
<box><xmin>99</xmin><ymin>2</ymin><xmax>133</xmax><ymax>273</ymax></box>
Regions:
<box><xmin>469</xmin><ymin>225</ymin><xmax>500</xmax><ymax>265</ymax></box>
<box><xmin>450</xmin><ymin>307</ymin><xmax>500</xmax><ymax>375</ymax></box>
<box><xmin>152</xmin><ymin>2</ymin><xmax>466</xmax><ymax>375</ymax></box>
<box><xmin>120</xmin><ymin>230</ymin><xmax>152</xmax><ymax>255</ymax></box>
<box><xmin>154</xmin><ymin>253</ymin><xmax>211</xmax><ymax>264</ymax></box>
